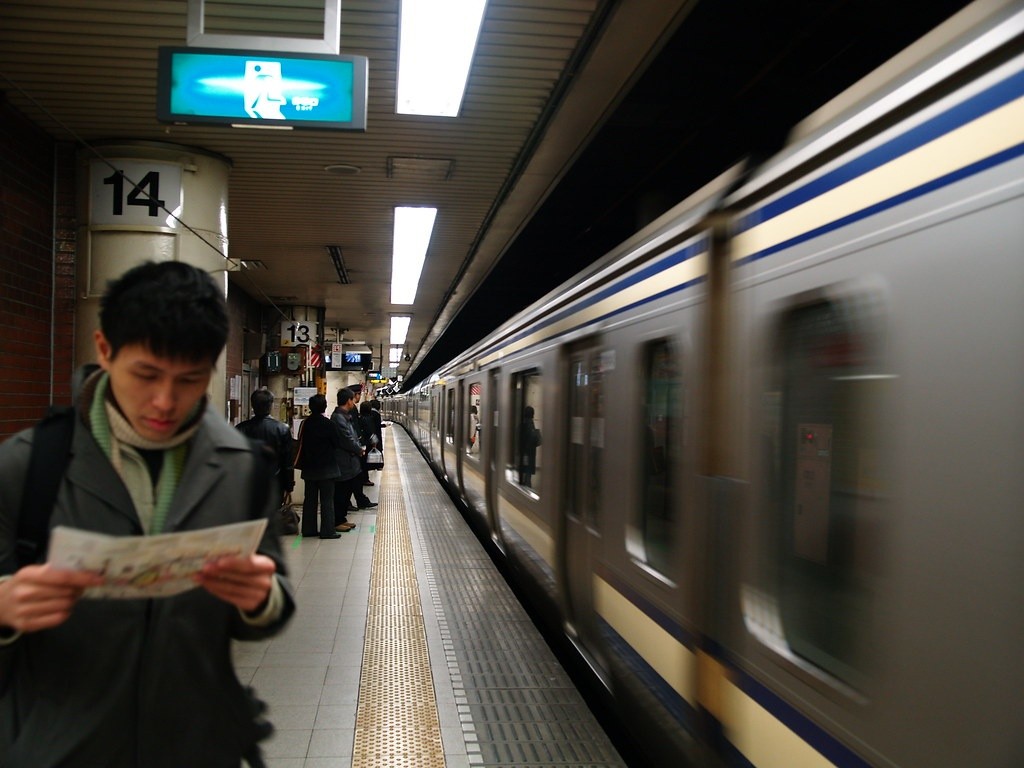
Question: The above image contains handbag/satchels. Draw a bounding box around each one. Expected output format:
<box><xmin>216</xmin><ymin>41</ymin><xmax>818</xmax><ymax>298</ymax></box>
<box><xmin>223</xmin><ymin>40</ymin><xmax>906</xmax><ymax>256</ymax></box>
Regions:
<box><xmin>535</xmin><ymin>428</ymin><xmax>544</xmax><ymax>448</ymax></box>
<box><xmin>293</xmin><ymin>420</ymin><xmax>306</xmax><ymax>468</ymax></box>
<box><xmin>471</xmin><ymin>435</ymin><xmax>477</xmax><ymax>444</ymax></box>
<box><xmin>366</xmin><ymin>447</ymin><xmax>384</xmax><ymax>463</ymax></box>
<box><xmin>274</xmin><ymin>492</ymin><xmax>301</xmax><ymax>537</ymax></box>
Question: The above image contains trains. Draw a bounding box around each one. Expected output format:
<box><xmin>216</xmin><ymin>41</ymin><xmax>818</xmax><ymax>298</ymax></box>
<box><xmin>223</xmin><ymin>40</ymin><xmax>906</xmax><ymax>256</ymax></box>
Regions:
<box><xmin>379</xmin><ymin>1</ymin><xmax>1024</xmax><ymax>768</ymax></box>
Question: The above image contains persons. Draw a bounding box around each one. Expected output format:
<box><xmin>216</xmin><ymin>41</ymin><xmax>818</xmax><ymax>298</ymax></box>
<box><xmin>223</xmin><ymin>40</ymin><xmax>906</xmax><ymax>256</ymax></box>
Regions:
<box><xmin>469</xmin><ymin>405</ymin><xmax>540</xmax><ymax>486</ymax></box>
<box><xmin>0</xmin><ymin>256</ymin><xmax>296</xmax><ymax>768</ymax></box>
<box><xmin>233</xmin><ymin>381</ymin><xmax>392</xmax><ymax>539</ymax></box>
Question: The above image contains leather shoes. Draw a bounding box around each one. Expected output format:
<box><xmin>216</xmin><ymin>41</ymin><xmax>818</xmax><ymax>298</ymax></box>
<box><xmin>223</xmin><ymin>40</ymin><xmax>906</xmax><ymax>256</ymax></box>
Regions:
<box><xmin>333</xmin><ymin>524</ymin><xmax>351</xmax><ymax>532</ymax></box>
<box><xmin>343</xmin><ymin>522</ymin><xmax>356</xmax><ymax>528</ymax></box>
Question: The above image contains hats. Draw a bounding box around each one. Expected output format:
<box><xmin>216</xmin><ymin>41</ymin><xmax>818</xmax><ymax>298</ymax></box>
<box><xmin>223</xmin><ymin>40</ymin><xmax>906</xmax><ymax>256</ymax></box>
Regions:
<box><xmin>348</xmin><ymin>384</ymin><xmax>364</xmax><ymax>393</ymax></box>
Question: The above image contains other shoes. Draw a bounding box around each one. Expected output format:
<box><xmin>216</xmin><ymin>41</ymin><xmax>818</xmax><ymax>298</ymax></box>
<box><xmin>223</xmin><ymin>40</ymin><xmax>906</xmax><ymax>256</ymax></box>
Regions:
<box><xmin>301</xmin><ymin>530</ymin><xmax>321</xmax><ymax>538</ymax></box>
<box><xmin>358</xmin><ymin>501</ymin><xmax>379</xmax><ymax>510</ymax></box>
<box><xmin>347</xmin><ymin>506</ymin><xmax>358</xmax><ymax>511</ymax></box>
<box><xmin>363</xmin><ymin>479</ymin><xmax>375</xmax><ymax>487</ymax></box>
<box><xmin>320</xmin><ymin>532</ymin><xmax>342</xmax><ymax>540</ymax></box>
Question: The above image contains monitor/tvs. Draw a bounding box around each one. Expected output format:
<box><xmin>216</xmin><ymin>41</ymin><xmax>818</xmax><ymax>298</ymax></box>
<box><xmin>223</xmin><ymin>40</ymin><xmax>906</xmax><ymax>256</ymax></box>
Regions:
<box><xmin>155</xmin><ymin>46</ymin><xmax>370</xmax><ymax>132</ymax></box>
<box><xmin>367</xmin><ymin>373</ymin><xmax>380</xmax><ymax>380</ymax></box>
<box><xmin>342</xmin><ymin>354</ymin><xmax>371</xmax><ymax>366</ymax></box>
